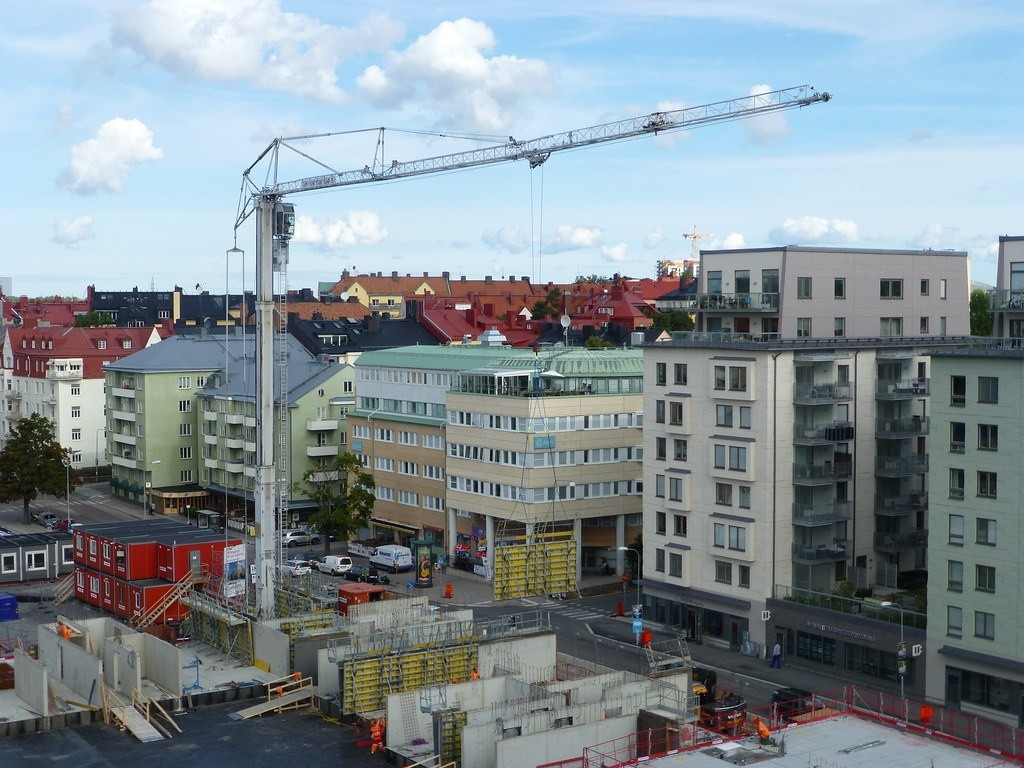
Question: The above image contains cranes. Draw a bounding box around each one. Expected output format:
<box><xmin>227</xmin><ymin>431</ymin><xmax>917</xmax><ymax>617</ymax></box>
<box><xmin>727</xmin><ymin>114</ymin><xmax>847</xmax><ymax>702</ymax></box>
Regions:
<box><xmin>226</xmin><ymin>85</ymin><xmax>834</xmax><ymax>620</ymax></box>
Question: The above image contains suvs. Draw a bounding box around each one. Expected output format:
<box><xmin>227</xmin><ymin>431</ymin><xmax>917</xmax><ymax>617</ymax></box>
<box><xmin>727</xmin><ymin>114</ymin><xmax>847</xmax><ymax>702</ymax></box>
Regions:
<box><xmin>281</xmin><ymin>530</ymin><xmax>320</xmax><ymax>548</ymax></box>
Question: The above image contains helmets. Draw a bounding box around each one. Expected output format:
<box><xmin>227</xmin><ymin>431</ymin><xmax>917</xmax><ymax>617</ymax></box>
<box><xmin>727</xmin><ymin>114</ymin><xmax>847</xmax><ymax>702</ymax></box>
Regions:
<box><xmin>370</xmin><ymin>719</ymin><xmax>376</xmax><ymax>724</ymax></box>
<box><xmin>452</xmin><ymin>679</ymin><xmax>457</xmax><ymax>683</ymax></box>
<box><xmin>752</xmin><ymin>716</ymin><xmax>757</xmax><ymax>723</ymax></box>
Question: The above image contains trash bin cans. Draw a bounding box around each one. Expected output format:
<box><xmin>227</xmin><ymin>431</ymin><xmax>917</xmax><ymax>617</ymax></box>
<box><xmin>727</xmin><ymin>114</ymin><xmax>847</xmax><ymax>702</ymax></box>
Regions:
<box><xmin>642</xmin><ymin>628</ymin><xmax>653</xmax><ymax>647</ymax></box>
<box><xmin>446</xmin><ymin>585</ymin><xmax>451</xmax><ymax>596</ymax></box>
<box><xmin>921</xmin><ymin>706</ymin><xmax>933</xmax><ymax>723</ymax></box>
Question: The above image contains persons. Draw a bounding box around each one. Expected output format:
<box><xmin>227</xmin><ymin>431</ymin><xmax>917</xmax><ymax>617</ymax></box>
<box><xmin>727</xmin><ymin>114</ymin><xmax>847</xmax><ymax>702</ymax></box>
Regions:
<box><xmin>769</xmin><ymin>642</ymin><xmax>781</xmax><ymax>669</ymax></box>
<box><xmin>59</xmin><ymin>622</ymin><xmax>69</xmax><ymax>640</ymax></box>
<box><xmin>752</xmin><ymin>714</ymin><xmax>770</xmax><ymax>745</ymax></box>
<box><xmin>370</xmin><ymin>718</ymin><xmax>385</xmax><ymax>754</ymax></box>
<box><xmin>451</xmin><ymin>668</ymin><xmax>480</xmax><ymax>684</ymax></box>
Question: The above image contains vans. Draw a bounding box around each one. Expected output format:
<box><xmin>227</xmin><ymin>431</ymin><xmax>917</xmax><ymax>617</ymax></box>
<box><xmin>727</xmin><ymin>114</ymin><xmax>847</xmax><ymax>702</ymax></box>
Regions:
<box><xmin>316</xmin><ymin>555</ymin><xmax>353</xmax><ymax>575</ymax></box>
<box><xmin>770</xmin><ymin>687</ymin><xmax>828</xmax><ymax>721</ymax></box>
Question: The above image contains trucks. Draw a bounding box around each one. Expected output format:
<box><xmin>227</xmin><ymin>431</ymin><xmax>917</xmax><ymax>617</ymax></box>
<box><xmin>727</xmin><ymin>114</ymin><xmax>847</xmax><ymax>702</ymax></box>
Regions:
<box><xmin>369</xmin><ymin>544</ymin><xmax>414</xmax><ymax>572</ymax></box>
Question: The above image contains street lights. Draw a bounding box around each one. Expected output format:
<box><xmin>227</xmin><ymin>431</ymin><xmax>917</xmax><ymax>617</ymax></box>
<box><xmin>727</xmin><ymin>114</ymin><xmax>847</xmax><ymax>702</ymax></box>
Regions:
<box><xmin>881</xmin><ymin>600</ymin><xmax>908</xmax><ymax>701</ymax></box>
<box><xmin>616</xmin><ymin>547</ymin><xmax>643</xmax><ymax>646</ymax></box>
<box><xmin>142</xmin><ymin>460</ymin><xmax>162</xmax><ymax>516</ymax></box>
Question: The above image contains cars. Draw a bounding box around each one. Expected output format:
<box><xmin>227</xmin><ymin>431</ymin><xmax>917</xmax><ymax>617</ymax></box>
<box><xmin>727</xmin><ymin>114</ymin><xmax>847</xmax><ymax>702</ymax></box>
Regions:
<box><xmin>58</xmin><ymin>518</ymin><xmax>77</xmax><ymax>532</ymax></box>
<box><xmin>38</xmin><ymin>512</ymin><xmax>60</xmax><ymax>528</ymax></box>
<box><xmin>68</xmin><ymin>523</ymin><xmax>84</xmax><ymax>535</ymax></box>
<box><xmin>290</xmin><ymin>552</ymin><xmax>321</xmax><ymax>569</ymax></box>
<box><xmin>343</xmin><ymin>566</ymin><xmax>379</xmax><ymax>583</ymax></box>
